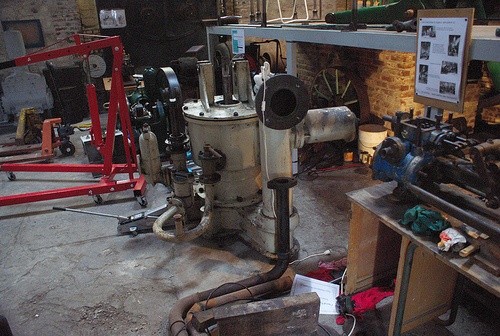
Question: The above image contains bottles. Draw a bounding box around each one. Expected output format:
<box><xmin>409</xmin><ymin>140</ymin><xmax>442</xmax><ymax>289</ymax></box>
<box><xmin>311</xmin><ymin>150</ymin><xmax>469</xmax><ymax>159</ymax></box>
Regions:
<box><xmin>139</xmin><ymin>126</ymin><xmax>161</xmax><ymax>175</ymax></box>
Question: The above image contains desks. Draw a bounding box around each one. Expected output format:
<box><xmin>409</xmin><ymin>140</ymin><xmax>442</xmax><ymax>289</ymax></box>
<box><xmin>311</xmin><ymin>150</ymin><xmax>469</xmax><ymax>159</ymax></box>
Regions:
<box><xmin>343</xmin><ymin>180</ymin><xmax>500</xmax><ymax>336</ymax></box>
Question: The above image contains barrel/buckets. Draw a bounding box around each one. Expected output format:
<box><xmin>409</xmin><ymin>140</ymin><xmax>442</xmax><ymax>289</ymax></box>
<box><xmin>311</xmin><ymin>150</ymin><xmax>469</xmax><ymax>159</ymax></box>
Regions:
<box><xmin>357</xmin><ymin>124</ymin><xmax>388</xmax><ymax>163</ymax></box>
